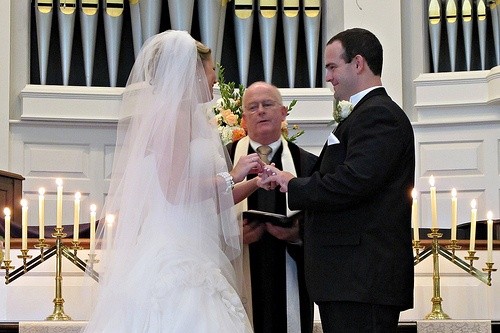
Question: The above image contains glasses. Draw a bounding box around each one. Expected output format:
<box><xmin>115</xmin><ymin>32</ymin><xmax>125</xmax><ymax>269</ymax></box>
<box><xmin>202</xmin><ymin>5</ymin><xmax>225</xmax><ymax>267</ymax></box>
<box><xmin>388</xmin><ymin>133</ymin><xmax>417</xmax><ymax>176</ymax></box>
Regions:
<box><xmin>245</xmin><ymin>99</ymin><xmax>281</xmax><ymax>115</ymax></box>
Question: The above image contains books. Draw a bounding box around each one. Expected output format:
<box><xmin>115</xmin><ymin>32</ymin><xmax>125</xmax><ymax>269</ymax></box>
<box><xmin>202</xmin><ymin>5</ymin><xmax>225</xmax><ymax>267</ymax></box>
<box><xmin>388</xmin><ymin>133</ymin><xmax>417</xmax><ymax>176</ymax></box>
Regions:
<box><xmin>239</xmin><ymin>207</ymin><xmax>302</xmax><ymax>226</ymax></box>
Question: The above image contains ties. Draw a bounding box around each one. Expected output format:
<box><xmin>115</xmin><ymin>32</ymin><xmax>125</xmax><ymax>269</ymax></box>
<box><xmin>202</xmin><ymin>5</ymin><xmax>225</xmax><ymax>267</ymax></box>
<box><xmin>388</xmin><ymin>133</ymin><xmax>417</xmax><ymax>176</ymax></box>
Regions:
<box><xmin>257</xmin><ymin>145</ymin><xmax>272</xmax><ymax>166</ymax></box>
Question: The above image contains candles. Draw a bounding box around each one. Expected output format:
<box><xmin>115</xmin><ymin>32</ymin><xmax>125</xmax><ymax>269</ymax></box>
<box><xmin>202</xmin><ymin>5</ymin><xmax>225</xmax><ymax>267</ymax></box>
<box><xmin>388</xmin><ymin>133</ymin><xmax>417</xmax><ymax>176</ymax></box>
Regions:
<box><xmin>430</xmin><ymin>187</ymin><xmax>437</xmax><ymax>228</ymax></box>
<box><xmin>5</xmin><ymin>215</ymin><xmax>11</xmax><ymax>260</ymax></box>
<box><xmin>108</xmin><ymin>224</ymin><xmax>112</xmax><ymax>249</ymax></box>
<box><xmin>487</xmin><ymin>221</ymin><xmax>493</xmax><ymax>263</ymax></box>
<box><xmin>90</xmin><ymin>212</ymin><xmax>96</xmax><ymax>254</ymax></box>
<box><xmin>470</xmin><ymin>208</ymin><xmax>477</xmax><ymax>250</ymax></box>
<box><xmin>412</xmin><ymin>200</ymin><xmax>419</xmax><ymax>240</ymax></box>
<box><xmin>39</xmin><ymin>196</ymin><xmax>45</xmax><ymax>239</ymax></box>
<box><xmin>22</xmin><ymin>206</ymin><xmax>28</xmax><ymax>250</ymax></box>
<box><xmin>451</xmin><ymin>197</ymin><xmax>458</xmax><ymax>240</ymax></box>
<box><xmin>73</xmin><ymin>199</ymin><xmax>79</xmax><ymax>242</ymax></box>
<box><xmin>57</xmin><ymin>186</ymin><xmax>62</xmax><ymax>228</ymax></box>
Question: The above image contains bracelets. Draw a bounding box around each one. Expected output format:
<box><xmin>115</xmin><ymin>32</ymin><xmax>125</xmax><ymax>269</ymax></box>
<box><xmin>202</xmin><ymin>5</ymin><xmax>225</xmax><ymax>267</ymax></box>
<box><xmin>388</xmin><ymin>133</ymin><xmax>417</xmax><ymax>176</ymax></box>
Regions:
<box><xmin>216</xmin><ymin>171</ymin><xmax>235</xmax><ymax>195</ymax></box>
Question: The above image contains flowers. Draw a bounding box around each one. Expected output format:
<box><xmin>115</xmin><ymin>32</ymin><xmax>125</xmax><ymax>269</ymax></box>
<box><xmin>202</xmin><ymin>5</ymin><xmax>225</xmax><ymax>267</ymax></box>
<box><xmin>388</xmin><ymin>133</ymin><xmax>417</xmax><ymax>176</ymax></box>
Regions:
<box><xmin>326</xmin><ymin>100</ymin><xmax>355</xmax><ymax>136</ymax></box>
<box><xmin>207</xmin><ymin>63</ymin><xmax>306</xmax><ymax>146</ymax></box>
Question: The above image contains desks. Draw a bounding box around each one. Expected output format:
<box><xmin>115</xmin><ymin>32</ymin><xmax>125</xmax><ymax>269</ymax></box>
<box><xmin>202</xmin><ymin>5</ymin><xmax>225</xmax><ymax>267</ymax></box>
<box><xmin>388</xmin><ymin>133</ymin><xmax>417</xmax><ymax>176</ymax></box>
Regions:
<box><xmin>0</xmin><ymin>320</ymin><xmax>500</xmax><ymax>333</ymax></box>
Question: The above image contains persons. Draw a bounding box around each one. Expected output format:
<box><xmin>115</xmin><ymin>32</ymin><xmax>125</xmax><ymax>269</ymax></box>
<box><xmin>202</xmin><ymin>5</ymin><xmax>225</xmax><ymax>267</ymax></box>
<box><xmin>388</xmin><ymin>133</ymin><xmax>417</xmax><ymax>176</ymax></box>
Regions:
<box><xmin>263</xmin><ymin>28</ymin><xmax>414</xmax><ymax>333</ymax></box>
<box><xmin>214</xmin><ymin>80</ymin><xmax>319</xmax><ymax>333</ymax></box>
<box><xmin>78</xmin><ymin>30</ymin><xmax>274</xmax><ymax>333</ymax></box>
